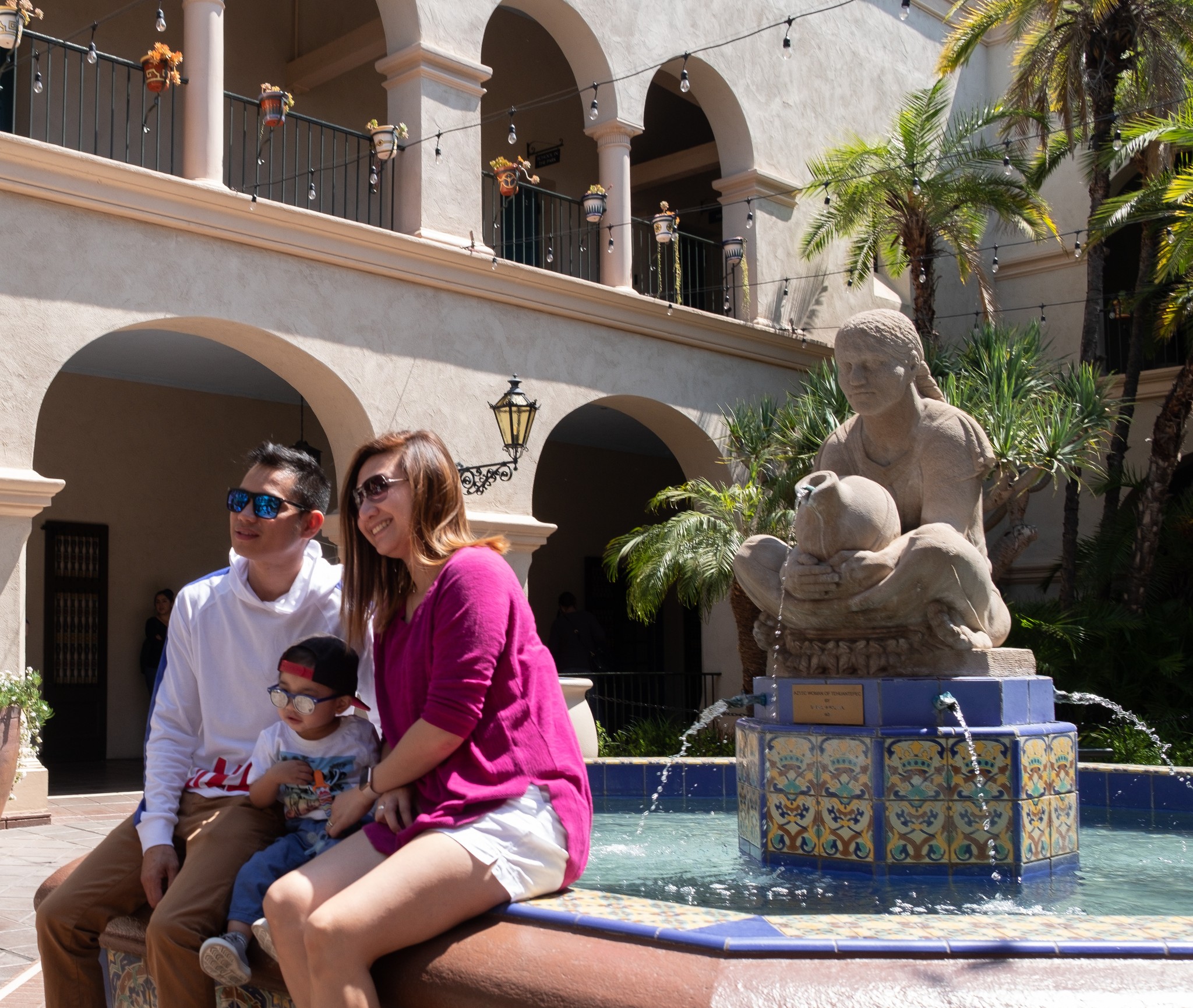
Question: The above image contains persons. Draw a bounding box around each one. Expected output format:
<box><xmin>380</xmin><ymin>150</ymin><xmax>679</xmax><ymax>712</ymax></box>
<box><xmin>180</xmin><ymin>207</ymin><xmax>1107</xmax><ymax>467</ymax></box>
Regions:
<box><xmin>141</xmin><ymin>588</ymin><xmax>177</xmax><ymax>699</ymax></box>
<box><xmin>35</xmin><ymin>440</ymin><xmax>382</xmax><ymax>1008</ymax></box>
<box><xmin>262</xmin><ymin>430</ymin><xmax>592</xmax><ymax>1008</ymax></box>
<box><xmin>199</xmin><ymin>635</ymin><xmax>382</xmax><ymax>986</ymax></box>
<box><xmin>732</xmin><ymin>309</ymin><xmax>1012</xmax><ymax>648</ymax></box>
<box><xmin>547</xmin><ymin>591</ymin><xmax>606</xmax><ymax>727</ymax></box>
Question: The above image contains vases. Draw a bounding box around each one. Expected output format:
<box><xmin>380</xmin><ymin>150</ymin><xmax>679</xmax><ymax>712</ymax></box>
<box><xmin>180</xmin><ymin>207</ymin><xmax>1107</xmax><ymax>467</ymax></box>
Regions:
<box><xmin>494</xmin><ymin>166</ymin><xmax>519</xmax><ymax>195</ymax></box>
<box><xmin>581</xmin><ymin>194</ymin><xmax>608</xmax><ymax>222</ymax></box>
<box><xmin>141</xmin><ymin>54</ymin><xmax>171</xmax><ymax>92</ymax></box>
<box><xmin>723</xmin><ymin>238</ymin><xmax>744</xmax><ymax>263</ymax></box>
<box><xmin>259</xmin><ymin>92</ymin><xmax>289</xmax><ymax>126</ymax></box>
<box><xmin>651</xmin><ymin>215</ymin><xmax>676</xmax><ymax>242</ymax></box>
<box><xmin>0</xmin><ymin>9</ymin><xmax>24</xmax><ymax>48</ymax></box>
<box><xmin>371</xmin><ymin>125</ymin><xmax>400</xmax><ymax>159</ymax></box>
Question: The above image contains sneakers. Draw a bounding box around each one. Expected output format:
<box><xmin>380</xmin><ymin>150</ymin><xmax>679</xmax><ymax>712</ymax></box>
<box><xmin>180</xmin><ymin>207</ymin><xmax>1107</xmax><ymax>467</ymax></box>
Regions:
<box><xmin>251</xmin><ymin>917</ymin><xmax>279</xmax><ymax>963</ymax></box>
<box><xmin>199</xmin><ymin>933</ymin><xmax>252</xmax><ymax>987</ymax></box>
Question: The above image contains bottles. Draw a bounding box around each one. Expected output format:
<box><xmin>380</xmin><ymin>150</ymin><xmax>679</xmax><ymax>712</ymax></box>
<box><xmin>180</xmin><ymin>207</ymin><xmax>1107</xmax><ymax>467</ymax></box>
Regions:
<box><xmin>793</xmin><ymin>471</ymin><xmax>900</xmax><ymax>557</ymax></box>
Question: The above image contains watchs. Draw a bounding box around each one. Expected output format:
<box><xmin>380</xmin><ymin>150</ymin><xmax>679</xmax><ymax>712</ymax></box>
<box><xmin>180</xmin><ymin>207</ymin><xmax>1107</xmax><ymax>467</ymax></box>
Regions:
<box><xmin>359</xmin><ymin>766</ymin><xmax>380</xmax><ymax>800</ymax></box>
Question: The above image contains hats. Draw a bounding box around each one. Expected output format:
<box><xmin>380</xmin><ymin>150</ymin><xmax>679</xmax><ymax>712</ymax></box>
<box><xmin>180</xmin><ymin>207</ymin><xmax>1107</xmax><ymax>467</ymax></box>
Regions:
<box><xmin>277</xmin><ymin>632</ymin><xmax>373</xmax><ymax>713</ymax></box>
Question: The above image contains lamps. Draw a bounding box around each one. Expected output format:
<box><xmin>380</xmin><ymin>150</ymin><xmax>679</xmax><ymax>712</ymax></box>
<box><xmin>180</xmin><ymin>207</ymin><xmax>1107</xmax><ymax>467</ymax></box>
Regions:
<box><xmin>456</xmin><ymin>373</ymin><xmax>542</xmax><ymax>496</ymax></box>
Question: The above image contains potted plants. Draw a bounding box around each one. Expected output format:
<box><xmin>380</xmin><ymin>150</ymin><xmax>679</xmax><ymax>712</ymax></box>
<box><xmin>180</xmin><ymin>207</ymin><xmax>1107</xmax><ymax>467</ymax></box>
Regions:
<box><xmin>368</xmin><ymin>119</ymin><xmax>409</xmax><ymax>140</ymax></box>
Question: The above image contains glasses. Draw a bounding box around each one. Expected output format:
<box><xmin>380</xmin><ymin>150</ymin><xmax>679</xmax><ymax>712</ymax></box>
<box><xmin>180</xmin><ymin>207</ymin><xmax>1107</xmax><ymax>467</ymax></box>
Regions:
<box><xmin>266</xmin><ymin>682</ymin><xmax>340</xmax><ymax>715</ymax></box>
<box><xmin>226</xmin><ymin>489</ymin><xmax>313</xmax><ymax>519</ymax></box>
<box><xmin>352</xmin><ymin>475</ymin><xmax>410</xmax><ymax>509</ymax></box>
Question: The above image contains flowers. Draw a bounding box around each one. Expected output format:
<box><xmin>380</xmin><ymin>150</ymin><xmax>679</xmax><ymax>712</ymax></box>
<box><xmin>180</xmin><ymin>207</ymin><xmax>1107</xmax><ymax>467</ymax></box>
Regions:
<box><xmin>259</xmin><ymin>82</ymin><xmax>295</xmax><ymax>115</ymax></box>
<box><xmin>148</xmin><ymin>41</ymin><xmax>184</xmax><ymax>85</ymax></box>
<box><xmin>490</xmin><ymin>156</ymin><xmax>540</xmax><ymax>184</ymax></box>
<box><xmin>5</xmin><ymin>0</ymin><xmax>44</xmax><ymax>25</ymax></box>
<box><xmin>653</xmin><ymin>200</ymin><xmax>681</xmax><ymax>227</ymax></box>
<box><xmin>584</xmin><ymin>183</ymin><xmax>613</xmax><ymax>194</ymax></box>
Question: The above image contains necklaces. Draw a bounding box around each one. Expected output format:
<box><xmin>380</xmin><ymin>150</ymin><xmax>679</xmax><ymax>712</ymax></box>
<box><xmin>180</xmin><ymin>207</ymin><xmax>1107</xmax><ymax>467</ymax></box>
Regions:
<box><xmin>411</xmin><ymin>593</ymin><xmax>415</xmax><ymax>614</ymax></box>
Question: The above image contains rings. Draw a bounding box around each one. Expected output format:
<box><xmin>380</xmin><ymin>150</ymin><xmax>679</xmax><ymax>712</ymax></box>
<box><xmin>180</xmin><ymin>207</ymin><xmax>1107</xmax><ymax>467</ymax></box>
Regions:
<box><xmin>378</xmin><ymin>805</ymin><xmax>384</xmax><ymax>809</ymax></box>
<box><xmin>327</xmin><ymin>819</ymin><xmax>334</xmax><ymax>828</ymax></box>
